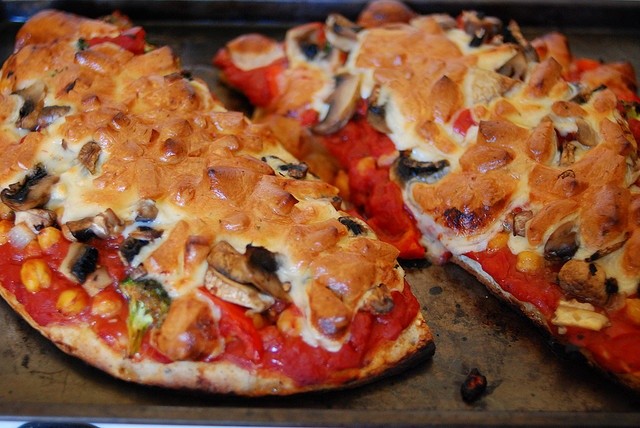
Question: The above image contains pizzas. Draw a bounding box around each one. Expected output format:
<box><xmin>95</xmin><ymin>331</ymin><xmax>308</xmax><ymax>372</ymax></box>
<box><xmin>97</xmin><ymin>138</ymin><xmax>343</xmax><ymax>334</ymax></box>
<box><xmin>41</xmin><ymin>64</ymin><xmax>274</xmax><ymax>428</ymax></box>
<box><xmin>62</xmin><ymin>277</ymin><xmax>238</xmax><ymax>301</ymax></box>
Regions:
<box><xmin>210</xmin><ymin>0</ymin><xmax>640</xmax><ymax>396</ymax></box>
<box><xmin>0</xmin><ymin>8</ymin><xmax>435</xmax><ymax>397</ymax></box>
